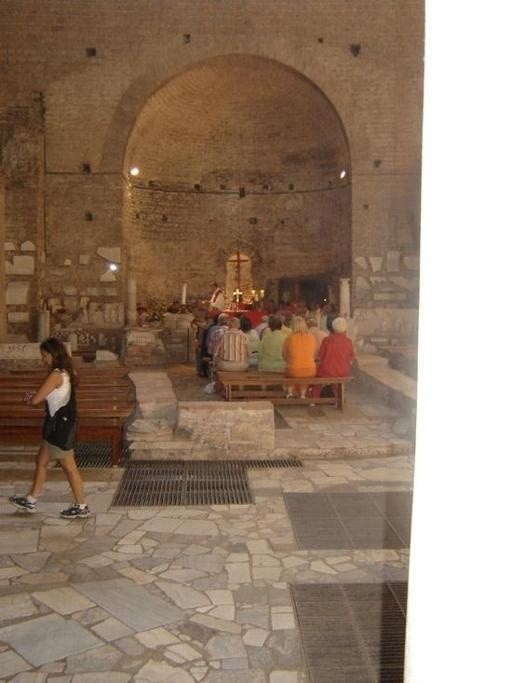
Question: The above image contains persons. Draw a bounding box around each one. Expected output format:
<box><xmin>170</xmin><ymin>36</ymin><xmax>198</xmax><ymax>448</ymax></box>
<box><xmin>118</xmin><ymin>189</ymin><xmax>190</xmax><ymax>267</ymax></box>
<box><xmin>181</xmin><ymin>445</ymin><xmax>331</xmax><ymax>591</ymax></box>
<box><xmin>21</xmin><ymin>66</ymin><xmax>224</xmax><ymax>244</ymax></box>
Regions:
<box><xmin>133</xmin><ymin>276</ymin><xmax>357</xmax><ymax>410</ymax></box>
<box><xmin>9</xmin><ymin>335</ymin><xmax>97</xmax><ymax>522</ymax></box>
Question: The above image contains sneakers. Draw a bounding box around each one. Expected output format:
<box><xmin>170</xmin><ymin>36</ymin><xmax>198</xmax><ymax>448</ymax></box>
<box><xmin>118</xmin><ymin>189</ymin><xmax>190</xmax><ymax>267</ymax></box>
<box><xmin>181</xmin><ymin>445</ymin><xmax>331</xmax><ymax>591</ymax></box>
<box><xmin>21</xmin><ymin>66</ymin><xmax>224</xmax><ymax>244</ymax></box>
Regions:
<box><xmin>9</xmin><ymin>496</ymin><xmax>35</xmax><ymax>513</ymax></box>
<box><xmin>59</xmin><ymin>503</ymin><xmax>90</xmax><ymax>518</ymax></box>
<box><xmin>285</xmin><ymin>393</ymin><xmax>305</xmax><ymax>398</ymax></box>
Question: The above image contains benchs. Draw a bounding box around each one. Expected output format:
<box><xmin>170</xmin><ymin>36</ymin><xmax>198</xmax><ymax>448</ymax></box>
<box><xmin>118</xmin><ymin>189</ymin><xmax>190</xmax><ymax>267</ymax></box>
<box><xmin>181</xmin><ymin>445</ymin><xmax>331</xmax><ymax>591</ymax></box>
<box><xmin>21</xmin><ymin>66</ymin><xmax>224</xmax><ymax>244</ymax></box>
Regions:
<box><xmin>0</xmin><ymin>367</ymin><xmax>135</xmax><ymax>465</ymax></box>
<box><xmin>204</xmin><ymin>357</ymin><xmax>355</xmax><ymax>411</ymax></box>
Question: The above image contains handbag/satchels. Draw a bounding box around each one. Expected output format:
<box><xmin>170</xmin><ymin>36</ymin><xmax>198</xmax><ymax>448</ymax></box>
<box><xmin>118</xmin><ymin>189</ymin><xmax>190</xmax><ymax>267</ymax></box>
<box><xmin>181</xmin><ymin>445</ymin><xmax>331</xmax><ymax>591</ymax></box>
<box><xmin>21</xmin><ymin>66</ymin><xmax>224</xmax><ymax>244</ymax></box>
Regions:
<box><xmin>42</xmin><ymin>415</ymin><xmax>78</xmax><ymax>449</ymax></box>
